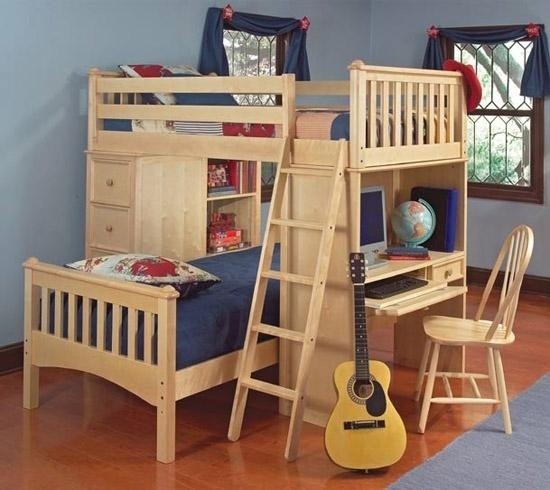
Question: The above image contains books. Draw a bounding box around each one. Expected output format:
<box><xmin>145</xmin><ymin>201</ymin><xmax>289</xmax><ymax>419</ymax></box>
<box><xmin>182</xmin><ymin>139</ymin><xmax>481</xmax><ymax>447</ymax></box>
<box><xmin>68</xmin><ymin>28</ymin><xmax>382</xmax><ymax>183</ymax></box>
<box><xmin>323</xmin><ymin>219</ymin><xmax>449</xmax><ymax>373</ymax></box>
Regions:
<box><xmin>385</xmin><ymin>247</ymin><xmax>430</xmax><ymax>256</ymax></box>
<box><xmin>387</xmin><ymin>254</ymin><xmax>432</xmax><ymax>261</ymax></box>
<box><xmin>209</xmin><ymin>241</ymin><xmax>247</xmax><ymax>254</ymax></box>
<box><xmin>208</xmin><ymin>158</ymin><xmax>257</xmax><ymax>197</ymax></box>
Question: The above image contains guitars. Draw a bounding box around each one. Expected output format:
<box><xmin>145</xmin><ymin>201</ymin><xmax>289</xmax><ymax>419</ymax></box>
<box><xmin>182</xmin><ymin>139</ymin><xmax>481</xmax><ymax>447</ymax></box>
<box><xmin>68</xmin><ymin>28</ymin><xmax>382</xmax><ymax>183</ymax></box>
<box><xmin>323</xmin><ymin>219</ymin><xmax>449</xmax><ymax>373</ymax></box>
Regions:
<box><xmin>325</xmin><ymin>254</ymin><xmax>407</xmax><ymax>471</ymax></box>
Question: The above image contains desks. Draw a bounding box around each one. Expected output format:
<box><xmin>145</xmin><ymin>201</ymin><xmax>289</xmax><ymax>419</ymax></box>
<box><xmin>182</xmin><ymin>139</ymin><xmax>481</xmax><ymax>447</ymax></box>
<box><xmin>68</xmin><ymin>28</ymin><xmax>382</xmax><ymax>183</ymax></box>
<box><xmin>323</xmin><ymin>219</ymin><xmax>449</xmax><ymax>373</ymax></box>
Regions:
<box><xmin>355</xmin><ymin>246</ymin><xmax>467</xmax><ymax>407</ymax></box>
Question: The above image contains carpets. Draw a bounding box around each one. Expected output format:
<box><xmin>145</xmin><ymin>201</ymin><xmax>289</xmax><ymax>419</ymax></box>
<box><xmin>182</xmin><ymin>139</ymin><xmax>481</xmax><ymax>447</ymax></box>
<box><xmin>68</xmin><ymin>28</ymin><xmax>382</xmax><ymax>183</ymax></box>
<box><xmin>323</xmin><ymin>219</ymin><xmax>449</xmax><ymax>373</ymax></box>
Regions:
<box><xmin>387</xmin><ymin>371</ymin><xmax>550</xmax><ymax>490</ymax></box>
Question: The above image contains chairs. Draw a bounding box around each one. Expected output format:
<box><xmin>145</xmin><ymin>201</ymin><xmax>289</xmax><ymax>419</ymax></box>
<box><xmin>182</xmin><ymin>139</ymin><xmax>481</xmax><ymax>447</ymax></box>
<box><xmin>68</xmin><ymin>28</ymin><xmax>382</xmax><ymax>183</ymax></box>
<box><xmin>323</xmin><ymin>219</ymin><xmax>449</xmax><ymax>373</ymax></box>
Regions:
<box><xmin>414</xmin><ymin>224</ymin><xmax>535</xmax><ymax>436</ymax></box>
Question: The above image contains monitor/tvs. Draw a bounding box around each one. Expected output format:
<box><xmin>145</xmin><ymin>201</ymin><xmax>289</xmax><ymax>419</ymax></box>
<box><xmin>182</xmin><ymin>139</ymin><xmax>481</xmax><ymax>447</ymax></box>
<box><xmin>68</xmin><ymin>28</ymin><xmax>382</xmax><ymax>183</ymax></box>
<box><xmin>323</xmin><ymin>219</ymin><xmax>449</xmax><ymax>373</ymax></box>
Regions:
<box><xmin>360</xmin><ymin>184</ymin><xmax>388</xmax><ymax>270</ymax></box>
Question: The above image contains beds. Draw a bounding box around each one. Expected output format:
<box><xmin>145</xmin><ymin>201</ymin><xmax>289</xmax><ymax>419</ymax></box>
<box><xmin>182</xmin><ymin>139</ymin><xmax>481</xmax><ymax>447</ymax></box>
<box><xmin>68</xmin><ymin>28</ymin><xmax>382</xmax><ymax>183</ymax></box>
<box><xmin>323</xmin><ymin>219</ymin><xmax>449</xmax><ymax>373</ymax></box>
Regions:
<box><xmin>87</xmin><ymin>57</ymin><xmax>468</xmax><ymax>171</ymax></box>
<box><xmin>22</xmin><ymin>243</ymin><xmax>280</xmax><ymax>464</ymax></box>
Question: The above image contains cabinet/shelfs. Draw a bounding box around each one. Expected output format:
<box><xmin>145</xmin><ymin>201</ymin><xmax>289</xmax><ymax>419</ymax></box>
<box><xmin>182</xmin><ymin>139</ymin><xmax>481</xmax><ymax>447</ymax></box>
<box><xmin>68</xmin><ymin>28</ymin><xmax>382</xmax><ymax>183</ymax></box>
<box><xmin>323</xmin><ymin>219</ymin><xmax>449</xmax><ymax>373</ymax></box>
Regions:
<box><xmin>207</xmin><ymin>159</ymin><xmax>259</xmax><ymax>258</ymax></box>
<box><xmin>83</xmin><ymin>152</ymin><xmax>207</xmax><ymax>263</ymax></box>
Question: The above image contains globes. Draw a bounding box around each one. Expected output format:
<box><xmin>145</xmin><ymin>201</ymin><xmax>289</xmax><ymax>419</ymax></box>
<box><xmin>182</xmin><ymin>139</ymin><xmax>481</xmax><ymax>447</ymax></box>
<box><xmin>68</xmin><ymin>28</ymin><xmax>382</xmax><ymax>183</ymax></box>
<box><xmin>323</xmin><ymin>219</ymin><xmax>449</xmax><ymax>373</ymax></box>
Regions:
<box><xmin>390</xmin><ymin>198</ymin><xmax>436</xmax><ymax>249</ymax></box>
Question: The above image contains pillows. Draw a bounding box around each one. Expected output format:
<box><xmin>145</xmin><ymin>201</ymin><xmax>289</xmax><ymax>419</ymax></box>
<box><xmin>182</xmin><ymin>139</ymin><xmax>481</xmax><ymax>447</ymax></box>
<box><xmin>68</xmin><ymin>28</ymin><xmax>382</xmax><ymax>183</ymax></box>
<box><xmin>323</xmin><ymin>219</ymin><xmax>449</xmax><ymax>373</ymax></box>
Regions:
<box><xmin>58</xmin><ymin>253</ymin><xmax>222</xmax><ymax>301</ymax></box>
<box><xmin>119</xmin><ymin>65</ymin><xmax>240</xmax><ymax>105</ymax></box>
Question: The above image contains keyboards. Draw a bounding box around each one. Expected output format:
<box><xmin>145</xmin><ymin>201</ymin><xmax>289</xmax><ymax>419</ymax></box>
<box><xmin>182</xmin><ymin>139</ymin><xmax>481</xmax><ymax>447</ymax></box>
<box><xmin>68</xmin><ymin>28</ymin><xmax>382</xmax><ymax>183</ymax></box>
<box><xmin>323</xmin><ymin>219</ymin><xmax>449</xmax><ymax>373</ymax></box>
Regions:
<box><xmin>365</xmin><ymin>275</ymin><xmax>428</xmax><ymax>299</ymax></box>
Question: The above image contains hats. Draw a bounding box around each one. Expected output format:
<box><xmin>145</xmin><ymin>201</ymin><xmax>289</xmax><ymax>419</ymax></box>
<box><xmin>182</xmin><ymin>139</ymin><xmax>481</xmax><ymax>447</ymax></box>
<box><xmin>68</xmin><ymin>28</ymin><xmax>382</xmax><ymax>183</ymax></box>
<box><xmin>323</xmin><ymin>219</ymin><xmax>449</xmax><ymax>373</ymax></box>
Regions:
<box><xmin>442</xmin><ymin>59</ymin><xmax>482</xmax><ymax>112</ymax></box>
